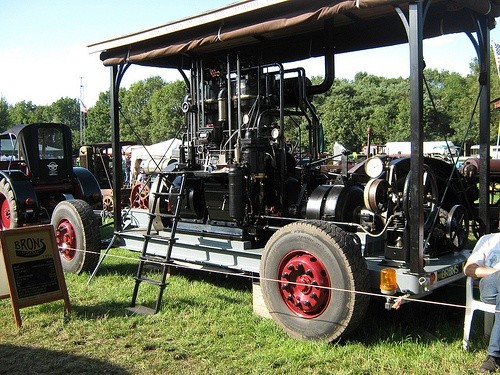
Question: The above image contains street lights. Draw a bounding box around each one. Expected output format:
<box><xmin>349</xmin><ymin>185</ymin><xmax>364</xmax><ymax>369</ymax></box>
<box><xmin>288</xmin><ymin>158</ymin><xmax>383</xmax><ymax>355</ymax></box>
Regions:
<box><xmin>366</xmin><ymin>127</ymin><xmax>372</xmax><ymax>158</ymax></box>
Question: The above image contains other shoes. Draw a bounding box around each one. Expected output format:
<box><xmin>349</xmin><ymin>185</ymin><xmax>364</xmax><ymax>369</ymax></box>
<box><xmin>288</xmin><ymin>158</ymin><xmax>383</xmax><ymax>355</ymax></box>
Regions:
<box><xmin>479</xmin><ymin>354</ymin><xmax>500</xmax><ymax>372</ymax></box>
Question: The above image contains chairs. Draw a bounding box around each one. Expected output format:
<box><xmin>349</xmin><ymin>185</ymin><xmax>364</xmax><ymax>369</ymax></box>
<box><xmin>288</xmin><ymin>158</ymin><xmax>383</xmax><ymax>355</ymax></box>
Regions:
<box><xmin>463</xmin><ymin>275</ymin><xmax>497</xmax><ymax>351</ymax></box>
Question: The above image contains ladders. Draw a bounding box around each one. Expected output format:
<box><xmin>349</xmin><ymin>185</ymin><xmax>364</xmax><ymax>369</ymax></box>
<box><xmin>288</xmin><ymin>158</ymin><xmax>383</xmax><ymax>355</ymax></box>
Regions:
<box><xmin>125</xmin><ymin>172</ymin><xmax>186</xmax><ymax>317</ymax></box>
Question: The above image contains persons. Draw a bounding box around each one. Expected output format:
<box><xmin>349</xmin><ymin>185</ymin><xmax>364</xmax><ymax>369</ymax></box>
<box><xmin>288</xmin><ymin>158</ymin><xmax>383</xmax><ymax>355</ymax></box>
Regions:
<box><xmin>461</xmin><ymin>216</ymin><xmax>498</xmax><ymax>369</ymax></box>
